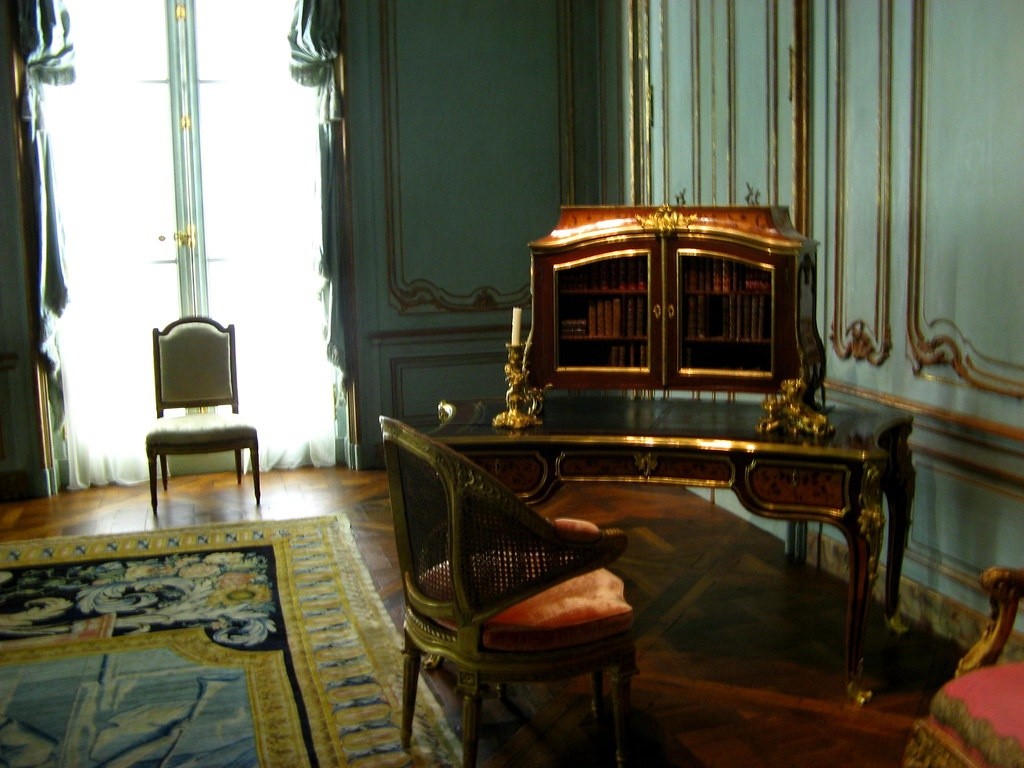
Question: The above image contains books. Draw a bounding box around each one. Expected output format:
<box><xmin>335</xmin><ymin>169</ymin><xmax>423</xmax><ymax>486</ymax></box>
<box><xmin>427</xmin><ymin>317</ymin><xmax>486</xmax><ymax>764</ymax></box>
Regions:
<box><xmin>680</xmin><ymin>255</ymin><xmax>772</xmax><ymax>372</ymax></box>
<box><xmin>558</xmin><ymin>256</ymin><xmax>649</xmax><ymax>366</ymax></box>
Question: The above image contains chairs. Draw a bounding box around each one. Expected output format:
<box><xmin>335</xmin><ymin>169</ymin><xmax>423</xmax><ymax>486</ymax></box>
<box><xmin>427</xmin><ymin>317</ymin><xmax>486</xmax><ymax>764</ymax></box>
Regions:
<box><xmin>900</xmin><ymin>567</ymin><xmax>1024</xmax><ymax>768</ymax></box>
<box><xmin>376</xmin><ymin>413</ymin><xmax>636</xmax><ymax>768</ymax></box>
<box><xmin>145</xmin><ymin>317</ymin><xmax>262</xmax><ymax>520</ymax></box>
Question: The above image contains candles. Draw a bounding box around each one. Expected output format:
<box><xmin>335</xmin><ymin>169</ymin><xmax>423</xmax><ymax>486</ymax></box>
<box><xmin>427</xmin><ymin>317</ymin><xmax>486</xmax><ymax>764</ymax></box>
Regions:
<box><xmin>510</xmin><ymin>304</ymin><xmax>521</xmax><ymax>347</ymax></box>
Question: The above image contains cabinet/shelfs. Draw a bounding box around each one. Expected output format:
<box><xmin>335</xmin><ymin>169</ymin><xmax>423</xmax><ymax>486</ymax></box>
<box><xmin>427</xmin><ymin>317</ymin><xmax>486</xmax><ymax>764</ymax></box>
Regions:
<box><xmin>520</xmin><ymin>205</ymin><xmax>835</xmax><ymax>416</ymax></box>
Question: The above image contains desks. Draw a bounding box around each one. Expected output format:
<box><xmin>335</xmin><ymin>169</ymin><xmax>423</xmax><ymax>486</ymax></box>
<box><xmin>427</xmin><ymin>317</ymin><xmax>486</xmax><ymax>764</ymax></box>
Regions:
<box><xmin>421</xmin><ymin>398</ymin><xmax>917</xmax><ymax>707</ymax></box>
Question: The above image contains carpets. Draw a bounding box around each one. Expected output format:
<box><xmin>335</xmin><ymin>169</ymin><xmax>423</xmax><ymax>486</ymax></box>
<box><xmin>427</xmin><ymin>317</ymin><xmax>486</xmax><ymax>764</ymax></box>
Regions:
<box><xmin>0</xmin><ymin>513</ymin><xmax>465</xmax><ymax>768</ymax></box>
